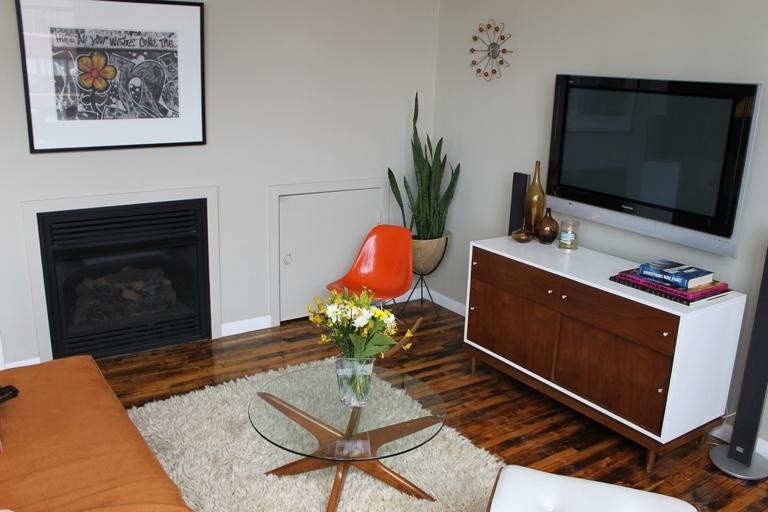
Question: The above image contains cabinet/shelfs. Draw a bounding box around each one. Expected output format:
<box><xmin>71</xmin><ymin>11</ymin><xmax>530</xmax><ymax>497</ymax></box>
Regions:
<box><xmin>463</xmin><ymin>233</ymin><xmax>748</xmax><ymax>453</ymax></box>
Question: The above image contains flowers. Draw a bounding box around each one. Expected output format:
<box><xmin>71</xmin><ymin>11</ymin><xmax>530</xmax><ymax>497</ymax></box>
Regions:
<box><xmin>311</xmin><ymin>286</ymin><xmax>404</xmax><ymax>363</ymax></box>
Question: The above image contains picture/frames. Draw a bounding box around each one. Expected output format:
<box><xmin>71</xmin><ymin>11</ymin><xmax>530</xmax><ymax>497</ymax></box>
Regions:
<box><xmin>16</xmin><ymin>0</ymin><xmax>208</xmax><ymax>155</ymax></box>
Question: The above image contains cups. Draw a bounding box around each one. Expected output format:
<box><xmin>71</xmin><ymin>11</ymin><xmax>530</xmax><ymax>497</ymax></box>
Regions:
<box><xmin>557</xmin><ymin>219</ymin><xmax>580</xmax><ymax>253</ymax></box>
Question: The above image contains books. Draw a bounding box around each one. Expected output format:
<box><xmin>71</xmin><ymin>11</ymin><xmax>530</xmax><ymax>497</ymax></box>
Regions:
<box><xmin>608</xmin><ymin>254</ymin><xmax>737</xmax><ymax>308</ymax></box>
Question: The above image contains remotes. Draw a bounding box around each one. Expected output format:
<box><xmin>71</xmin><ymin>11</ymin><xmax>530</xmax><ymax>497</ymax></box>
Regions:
<box><xmin>0</xmin><ymin>384</ymin><xmax>20</xmax><ymax>402</ymax></box>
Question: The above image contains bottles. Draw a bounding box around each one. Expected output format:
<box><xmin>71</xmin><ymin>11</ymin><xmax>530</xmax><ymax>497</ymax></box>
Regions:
<box><xmin>511</xmin><ymin>160</ymin><xmax>559</xmax><ymax>245</ymax></box>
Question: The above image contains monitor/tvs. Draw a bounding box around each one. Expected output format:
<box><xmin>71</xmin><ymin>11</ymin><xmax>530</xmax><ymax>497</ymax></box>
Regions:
<box><xmin>544</xmin><ymin>74</ymin><xmax>763</xmax><ymax>259</ymax></box>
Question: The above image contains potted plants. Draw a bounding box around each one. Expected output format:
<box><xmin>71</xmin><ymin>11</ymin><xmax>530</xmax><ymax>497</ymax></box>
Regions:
<box><xmin>388</xmin><ymin>91</ymin><xmax>460</xmax><ymax>274</ymax></box>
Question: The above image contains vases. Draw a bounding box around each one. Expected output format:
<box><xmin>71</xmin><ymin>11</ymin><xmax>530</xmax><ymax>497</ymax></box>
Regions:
<box><xmin>334</xmin><ymin>350</ymin><xmax>373</xmax><ymax>406</ymax></box>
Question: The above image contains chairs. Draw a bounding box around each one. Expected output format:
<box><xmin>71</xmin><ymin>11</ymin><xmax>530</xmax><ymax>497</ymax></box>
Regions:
<box><xmin>329</xmin><ymin>223</ymin><xmax>423</xmax><ymax>359</ymax></box>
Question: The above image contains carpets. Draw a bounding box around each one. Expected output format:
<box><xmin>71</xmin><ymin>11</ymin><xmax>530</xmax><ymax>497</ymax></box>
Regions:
<box><xmin>130</xmin><ymin>357</ymin><xmax>505</xmax><ymax>511</ymax></box>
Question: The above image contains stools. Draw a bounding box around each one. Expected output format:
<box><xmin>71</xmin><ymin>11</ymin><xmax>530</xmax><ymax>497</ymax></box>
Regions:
<box><xmin>491</xmin><ymin>460</ymin><xmax>695</xmax><ymax>512</ymax></box>
